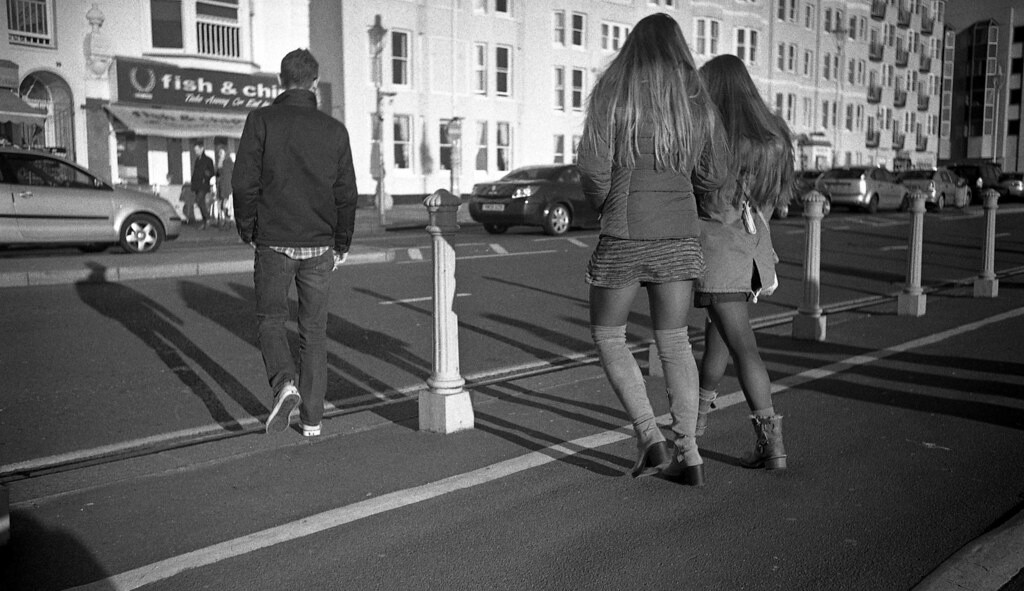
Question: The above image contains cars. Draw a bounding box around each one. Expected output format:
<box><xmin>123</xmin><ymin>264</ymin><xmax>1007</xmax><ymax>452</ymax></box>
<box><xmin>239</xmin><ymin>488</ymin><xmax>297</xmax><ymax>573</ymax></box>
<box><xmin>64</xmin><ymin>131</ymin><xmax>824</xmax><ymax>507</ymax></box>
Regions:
<box><xmin>895</xmin><ymin>167</ymin><xmax>973</xmax><ymax>213</ymax></box>
<box><xmin>813</xmin><ymin>166</ymin><xmax>911</xmax><ymax>214</ymax></box>
<box><xmin>770</xmin><ymin>170</ymin><xmax>828</xmax><ymax>222</ymax></box>
<box><xmin>467</xmin><ymin>164</ymin><xmax>603</xmax><ymax>237</ymax></box>
<box><xmin>0</xmin><ymin>147</ymin><xmax>181</xmax><ymax>256</ymax></box>
<box><xmin>1001</xmin><ymin>172</ymin><xmax>1024</xmax><ymax>201</ymax></box>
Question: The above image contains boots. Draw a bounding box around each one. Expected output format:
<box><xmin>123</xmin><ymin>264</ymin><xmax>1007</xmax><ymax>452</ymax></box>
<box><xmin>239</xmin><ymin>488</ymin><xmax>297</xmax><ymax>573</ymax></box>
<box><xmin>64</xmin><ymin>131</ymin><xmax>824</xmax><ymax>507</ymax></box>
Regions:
<box><xmin>653</xmin><ymin>326</ymin><xmax>704</xmax><ymax>486</ymax></box>
<box><xmin>740</xmin><ymin>415</ymin><xmax>787</xmax><ymax>469</ymax></box>
<box><xmin>696</xmin><ymin>396</ymin><xmax>715</xmax><ymax>437</ymax></box>
<box><xmin>588</xmin><ymin>324</ymin><xmax>670</xmax><ymax>478</ymax></box>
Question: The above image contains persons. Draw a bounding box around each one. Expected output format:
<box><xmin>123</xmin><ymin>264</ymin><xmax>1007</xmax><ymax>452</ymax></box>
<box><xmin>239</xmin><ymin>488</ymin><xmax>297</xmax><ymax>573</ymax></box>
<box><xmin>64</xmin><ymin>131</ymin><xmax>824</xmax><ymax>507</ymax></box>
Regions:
<box><xmin>575</xmin><ymin>11</ymin><xmax>794</xmax><ymax>488</ymax></box>
<box><xmin>189</xmin><ymin>141</ymin><xmax>235</xmax><ymax>231</ymax></box>
<box><xmin>228</xmin><ymin>48</ymin><xmax>359</xmax><ymax>436</ymax></box>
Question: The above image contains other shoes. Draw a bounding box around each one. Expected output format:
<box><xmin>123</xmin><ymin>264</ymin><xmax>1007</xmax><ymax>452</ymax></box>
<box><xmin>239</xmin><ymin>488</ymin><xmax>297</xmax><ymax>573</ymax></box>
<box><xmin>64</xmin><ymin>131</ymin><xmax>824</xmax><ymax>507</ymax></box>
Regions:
<box><xmin>205</xmin><ymin>219</ymin><xmax>211</xmax><ymax>229</ymax></box>
<box><xmin>197</xmin><ymin>222</ymin><xmax>205</xmax><ymax>229</ymax></box>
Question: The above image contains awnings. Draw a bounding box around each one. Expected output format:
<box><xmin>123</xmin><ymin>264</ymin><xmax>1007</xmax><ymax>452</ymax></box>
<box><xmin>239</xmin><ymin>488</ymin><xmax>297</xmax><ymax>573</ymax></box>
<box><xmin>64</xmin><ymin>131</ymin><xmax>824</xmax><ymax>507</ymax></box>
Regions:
<box><xmin>0</xmin><ymin>87</ymin><xmax>48</xmax><ymax>129</ymax></box>
<box><xmin>101</xmin><ymin>104</ymin><xmax>248</xmax><ymax>139</ymax></box>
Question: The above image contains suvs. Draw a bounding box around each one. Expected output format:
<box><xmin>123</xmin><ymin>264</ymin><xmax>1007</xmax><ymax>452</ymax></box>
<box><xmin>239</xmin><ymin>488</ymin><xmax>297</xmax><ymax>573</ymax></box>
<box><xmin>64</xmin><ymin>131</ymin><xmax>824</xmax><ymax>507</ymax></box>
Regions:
<box><xmin>949</xmin><ymin>158</ymin><xmax>1010</xmax><ymax>201</ymax></box>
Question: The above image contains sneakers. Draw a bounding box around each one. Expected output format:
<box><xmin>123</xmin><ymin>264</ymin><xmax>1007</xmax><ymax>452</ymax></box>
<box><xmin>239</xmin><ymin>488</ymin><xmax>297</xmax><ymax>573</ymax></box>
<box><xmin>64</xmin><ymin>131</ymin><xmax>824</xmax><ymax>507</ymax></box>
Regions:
<box><xmin>266</xmin><ymin>385</ymin><xmax>300</xmax><ymax>434</ymax></box>
<box><xmin>298</xmin><ymin>418</ymin><xmax>322</xmax><ymax>436</ymax></box>
